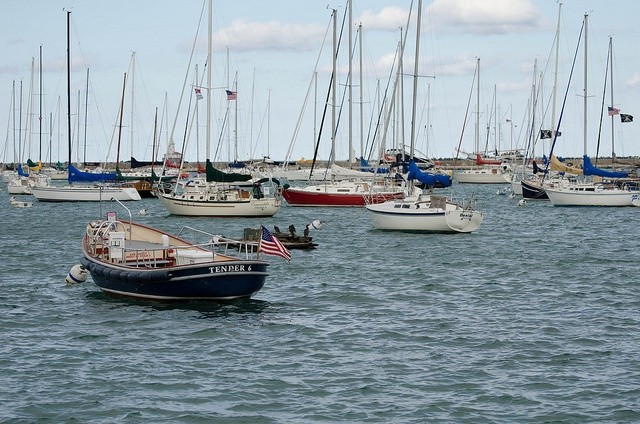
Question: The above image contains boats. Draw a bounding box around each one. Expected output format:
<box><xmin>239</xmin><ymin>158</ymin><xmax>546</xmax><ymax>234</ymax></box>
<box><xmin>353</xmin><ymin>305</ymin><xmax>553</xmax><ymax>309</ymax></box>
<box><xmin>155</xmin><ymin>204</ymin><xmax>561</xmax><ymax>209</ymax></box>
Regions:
<box><xmin>30</xmin><ymin>10</ymin><xmax>144</xmax><ymax>203</ymax></box>
<box><xmin>375</xmin><ymin>1</ymin><xmax>438</xmax><ymax>169</ymax></box>
<box><xmin>61</xmin><ymin>184</ymin><xmax>295</xmax><ymax>307</ymax></box>
<box><xmin>328</xmin><ymin>0</ymin><xmax>454</xmax><ymax>189</ymax></box>
<box><xmin>6</xmin><ymin>56</ymin><xmax>54</xmax><ymax>196</ymax></box>
<box><xmin>183</xmin><ymin>1</ymin><xmax>291</xmax><ymax>197</ymax></box>
<box><xmin>40</xmin><ymin>92</ymin><xmax>68</xmax><ymax>181</ymax></box>
<box><xmin>544</xmin><ymin>11</ymin><xmax>640</xmax><ymax>209</ymax></box>
<box><xmin>467</xmin><ymin>84</ymin><xmax>528</xmax><ymax>166</ymax></box>
<box><xmin>280</xmin><ymin>0</ymin><xmax>407</xmax><ymax>210</ymax></box>
<box><xmin>510</xmin><ymin>2</ymin><xmax>571</xmax><ymax>196</ymax></box>
<box><xmin>64</xmin><ymin>11</ymin><xmax>181</xmax><ymax>194</ymax></box>
<box><xmin>158</xmin><ymin>1</ymin><xmax>281</xmax><ymax>220</ymax></box>
<box><xmin>2</xmin><ymin>79</ymin><xmax>22</xmax><ymax>183</ymax></box>
<box><xmin>9</xmin><ymin>197</ymin><xmax>34</xmax><ymax>210</ymax></box>
<box><xmin>361</xmin><ymin>1</ymin><xmax>486</xmax><ymax>235</ymax></box>
<box><xmin>456</xmin><ymin>56</ymin><xmax>518</xmax><ymax>184</ymax></box>
<box><xmin>210</xmin><ymin>218</ymin><xmax>322</xmax><ymax>253</ymax></box>
<box><xmin>520</xmin><ymin>13</ymin><xmax>596</xmax><ymax>199</ymax></box>
<box><xmin>113</xmin><ymin>49</ymin><xmax>191</xmax><ymax>180</ymax></box>
